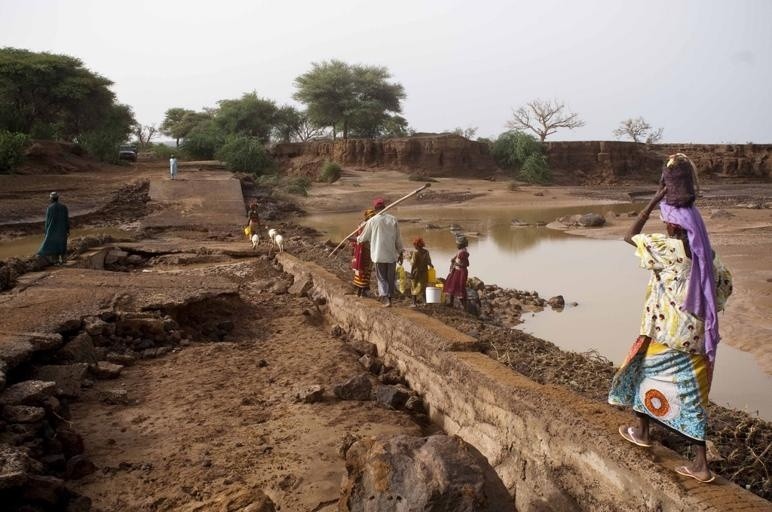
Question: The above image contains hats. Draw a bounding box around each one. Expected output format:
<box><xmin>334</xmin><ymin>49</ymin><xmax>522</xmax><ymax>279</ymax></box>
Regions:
<box><xmin>363</xmin><ymin>198</ymin><xmax>387</xmax><ymax>221</ymax></box>
<box><xmin>455</xmin><ymin>235</ymin><xmax>467</xmax><ymax>244</ymax></box>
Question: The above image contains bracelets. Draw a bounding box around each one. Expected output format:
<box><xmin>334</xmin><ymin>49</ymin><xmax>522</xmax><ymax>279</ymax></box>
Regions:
<box><xmin>639</xmin><ymin>210</ymin><xmax>650</xmax><ymax>222</ymax></box>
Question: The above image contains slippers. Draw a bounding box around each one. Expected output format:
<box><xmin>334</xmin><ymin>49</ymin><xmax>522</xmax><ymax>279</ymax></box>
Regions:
<box><xmin>674</xmin><ymin>465</ymin><xmax>716</xmax><ymax>483</ymax></box>
<box><xmin>618</xmin><ymin>424</ymin><xmax>654</xmax><ymax>448</ymax></box>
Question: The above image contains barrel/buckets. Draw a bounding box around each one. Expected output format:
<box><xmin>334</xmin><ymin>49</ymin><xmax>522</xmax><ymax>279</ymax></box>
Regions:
<box><xmin>428</xmin><ymin>266</ymin><xmax>436</xmax><ymax>283</ymax></box>
<box><xmin>425</xmin><ymin>287</ymin><xmax>443</xmax><ymax>304</ymax></box>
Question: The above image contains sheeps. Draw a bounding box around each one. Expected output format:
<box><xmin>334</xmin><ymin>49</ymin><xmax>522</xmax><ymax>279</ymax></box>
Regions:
<box><xmin>252</xmin><ymin>234</ymin><xmax>259</xmax><ymax>249</ymax></box>
<box><xmin>264</xmin><ymin>224</ymin><xmax>279</xmax><ymax>248</ymax></box>
<box><xmin>276</xmin><ymin>234</ymin><xmax>284</xmax><ymax>253</ymax></box>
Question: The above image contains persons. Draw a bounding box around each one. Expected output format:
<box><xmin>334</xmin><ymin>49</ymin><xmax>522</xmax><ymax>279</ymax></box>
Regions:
<box><xmin>606</xmin><ymin>173</ymin><xmax>722</xmax><ymax>483</ymax></box>
<box><xmin>347</xmin><ymin>198</ymin><xmax>405</xmax><ymax>308</ymax></box>
<box><xmin>408</xmin><ymin>238</ymin><xmax>434</xmax><ymax>307</ymax></box>
<box><xmin>350</xmin><ymin>224</ymin><xmax>373</xmax><ymax>298</ymax></box>
<box><xmin>442</xmin><ymin>236</ymin><xmax>470</xmax><ymax>311</ymax></box>
<box><xmin>170</xmin><ymin>155</ymin><xmax>177</xmax><ymax>180</ymax></box>
<box><xmin>37</xmin><ymin>192</ymin><xmax>68</xmax><ymax>264</ymax></box>
<box><xmin>244</xmin><ymin>203</ymin><xmax>260</xmax><ymax>241</ymax></box>
